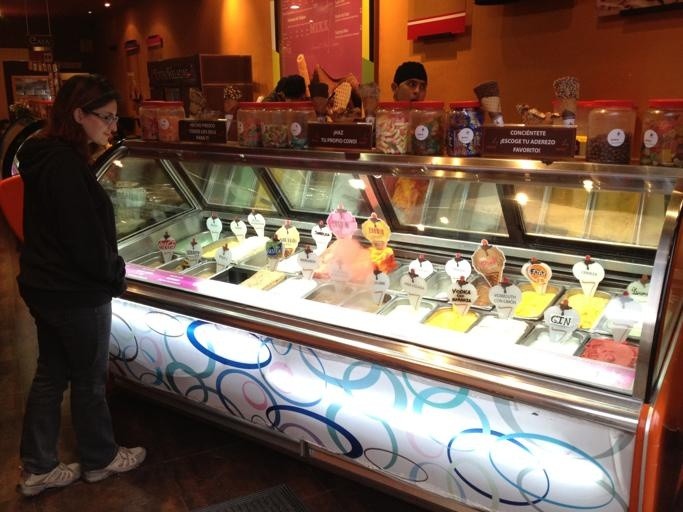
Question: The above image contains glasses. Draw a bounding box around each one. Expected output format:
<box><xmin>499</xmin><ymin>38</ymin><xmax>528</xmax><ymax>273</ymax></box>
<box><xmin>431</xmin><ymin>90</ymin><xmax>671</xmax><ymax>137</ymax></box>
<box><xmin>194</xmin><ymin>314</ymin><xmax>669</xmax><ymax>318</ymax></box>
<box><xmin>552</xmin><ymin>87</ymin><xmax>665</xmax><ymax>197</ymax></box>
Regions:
<box><xmin>88</xmin><ymin>110</ymin><xmax>119</xmax><ymax>126</ymax></box>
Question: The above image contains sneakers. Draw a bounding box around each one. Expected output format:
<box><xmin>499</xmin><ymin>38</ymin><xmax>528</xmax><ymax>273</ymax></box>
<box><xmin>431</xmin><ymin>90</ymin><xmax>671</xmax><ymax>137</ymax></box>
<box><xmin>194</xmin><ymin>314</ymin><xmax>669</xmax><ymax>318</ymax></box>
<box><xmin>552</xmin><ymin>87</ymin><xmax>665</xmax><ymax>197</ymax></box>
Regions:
<box><xmin>15</xmin><ymin>463</ymin><xmax>80</xmax><ymax>497</ymax></box>
<box><xmin>84</xmin><ymin>446</ymin><xmax>147</xmax><ymax>483</ymax></box>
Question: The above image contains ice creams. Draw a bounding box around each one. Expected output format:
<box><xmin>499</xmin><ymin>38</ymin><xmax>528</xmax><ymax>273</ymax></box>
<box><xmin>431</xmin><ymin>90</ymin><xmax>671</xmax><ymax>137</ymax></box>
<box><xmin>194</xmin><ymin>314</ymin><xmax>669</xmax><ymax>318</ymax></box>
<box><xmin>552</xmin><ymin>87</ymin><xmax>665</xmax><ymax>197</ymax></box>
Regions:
<box><xmin>361</xmin><ymin>213</ymin><xmax>391</xmax><ymax>251</ymax></box>
<box><xmin>472</xmin><ymin>239</ymin><xmax>505</xmax><ymax>287</ymax></box>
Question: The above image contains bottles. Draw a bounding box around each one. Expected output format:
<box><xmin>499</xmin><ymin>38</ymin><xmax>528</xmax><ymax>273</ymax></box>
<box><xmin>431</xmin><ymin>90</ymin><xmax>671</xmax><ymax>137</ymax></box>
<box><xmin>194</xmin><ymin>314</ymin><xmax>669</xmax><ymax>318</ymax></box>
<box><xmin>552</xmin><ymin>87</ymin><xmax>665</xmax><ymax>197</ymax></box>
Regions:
<box><xmin>236</xmin><ymin>101</ymin><xmax>317</xmax><ymax>149</ymax></box>
<box><xmin>138</xmin><ymin>99</ymin><xmax>185</xmax><ymax>143</ymax></box>
<box><xmin>374</xmin><ymin>100</ymin><xmax>481</xmax><ymax>157</ymax></box>
<box><xmin>584</xmin><ymin>98</ymin><xmax>681</xmax><ymax>168</ymax></box>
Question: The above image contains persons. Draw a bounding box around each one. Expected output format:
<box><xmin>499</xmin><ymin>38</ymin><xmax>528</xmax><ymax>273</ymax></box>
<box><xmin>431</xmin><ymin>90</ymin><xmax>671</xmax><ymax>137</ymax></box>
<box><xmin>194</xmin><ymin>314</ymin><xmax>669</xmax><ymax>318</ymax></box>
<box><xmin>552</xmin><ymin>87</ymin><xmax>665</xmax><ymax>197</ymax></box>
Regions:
<box><xmin>388</xmin><ymin>61</ymin><xmax>428</xmax><ymax>102</ymax></box>
<box><xmin>13</xmin><ymin>72</ymin><xmax>151</xmax><ymax>499</ymax></box>
<box><xmin>259</xmin><ymin>72</ymin><xmax>305</xmax><ymax>102</ymax></box>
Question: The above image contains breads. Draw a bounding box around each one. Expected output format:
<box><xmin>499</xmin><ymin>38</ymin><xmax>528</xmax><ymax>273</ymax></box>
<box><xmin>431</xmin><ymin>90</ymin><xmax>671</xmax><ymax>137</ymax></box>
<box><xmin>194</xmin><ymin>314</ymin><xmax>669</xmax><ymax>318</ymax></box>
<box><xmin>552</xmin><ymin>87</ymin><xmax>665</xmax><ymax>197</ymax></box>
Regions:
<box><xmin>141</xmin><ymin>183</ymin><xmax>183</xmax><ymax>218</ymax></box>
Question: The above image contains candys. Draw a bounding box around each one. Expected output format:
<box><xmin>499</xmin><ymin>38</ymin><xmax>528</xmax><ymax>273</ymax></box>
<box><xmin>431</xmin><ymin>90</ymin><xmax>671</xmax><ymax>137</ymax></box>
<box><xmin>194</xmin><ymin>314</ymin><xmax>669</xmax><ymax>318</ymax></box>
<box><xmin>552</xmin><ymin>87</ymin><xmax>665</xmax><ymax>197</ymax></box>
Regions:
<box><xmin>641</xmin><ymin>116</ymin><xmax>683</xmax><ymax>167</ymax></box>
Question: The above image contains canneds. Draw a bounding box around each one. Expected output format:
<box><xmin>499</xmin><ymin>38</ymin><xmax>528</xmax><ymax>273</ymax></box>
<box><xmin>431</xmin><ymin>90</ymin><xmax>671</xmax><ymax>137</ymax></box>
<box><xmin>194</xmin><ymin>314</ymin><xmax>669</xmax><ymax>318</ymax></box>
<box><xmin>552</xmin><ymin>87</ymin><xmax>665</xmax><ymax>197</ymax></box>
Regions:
<box><xmin>640</xmin><ymin>99</ymin><xmax>682</xmax><ymax>169</ymax></box>
<box><xmin>235</xmin><ymin>101</ymin><xmax>316</xmax><ymax>150</ymax></box>
<box><xmin>586</xmin><ymin>100</ymin><xmax>637</xmax><ymax>164</ymax></box>
<box><xmin>374</xmin><ymin>101</ymin><xmax>485</xmax><ymax>158</ymax></box>
<box><xmin>137</xmin><ymin>100</ymin><xmax>185</xmax><ymax>142</ymax></box>
